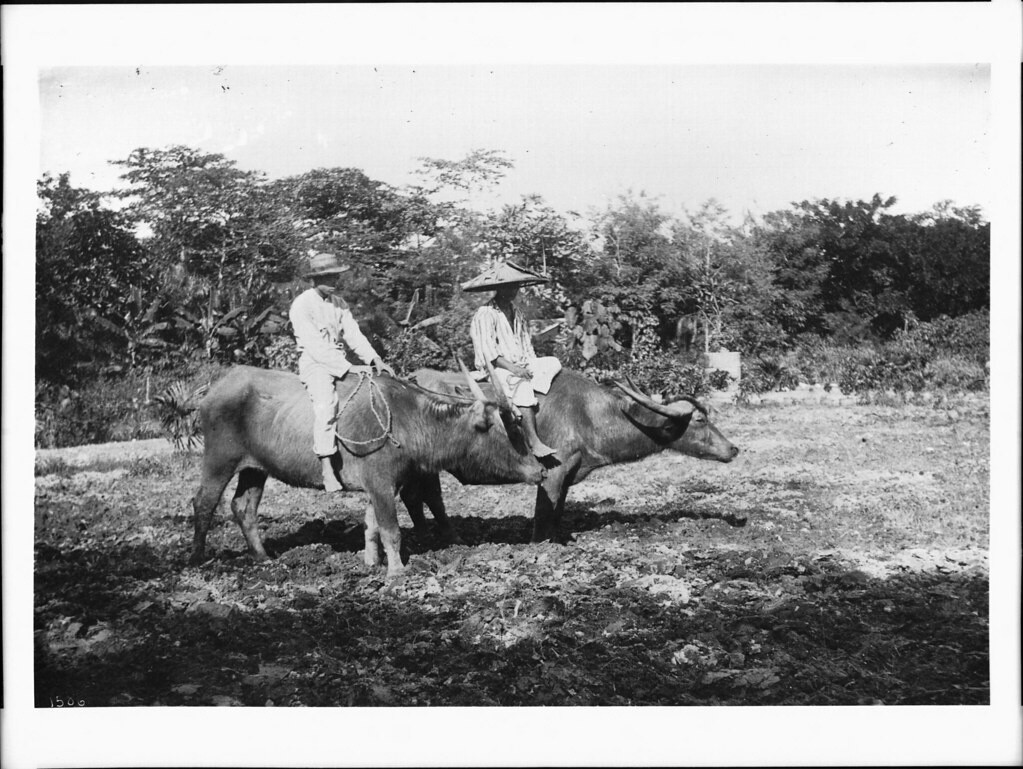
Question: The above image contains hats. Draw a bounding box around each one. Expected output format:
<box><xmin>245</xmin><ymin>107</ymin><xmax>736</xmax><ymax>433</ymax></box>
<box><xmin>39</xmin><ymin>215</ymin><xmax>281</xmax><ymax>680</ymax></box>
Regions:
<box><xmin>462</xmin><ymin>259</ymin><xmax>549</xmax><ymax>293</ymax></box>
<box><xmin>301</xmin><ymin>253</ymin><xmax>352</xmax><ymax>283</ymax></box>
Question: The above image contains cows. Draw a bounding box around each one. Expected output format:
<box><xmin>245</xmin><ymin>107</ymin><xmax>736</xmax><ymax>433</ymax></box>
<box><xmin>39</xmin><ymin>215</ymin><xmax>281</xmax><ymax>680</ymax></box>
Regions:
<box><xmin>190</xmin><ymin>367</ymin><xmax>738</xmax><ymax>575</ymax></box>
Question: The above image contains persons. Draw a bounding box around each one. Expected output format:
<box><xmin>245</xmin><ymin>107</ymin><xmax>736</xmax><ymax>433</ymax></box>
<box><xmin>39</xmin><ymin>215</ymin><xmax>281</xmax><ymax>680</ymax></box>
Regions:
<box><xmin>289</xmin><ymin>253</ymin><xmax>399</xmax><ymax>493</ymax></box>
<box><xmin>470</xmin><ymin>277</ymin><xmax>558</xmax><ymax>458</ymax></box>
